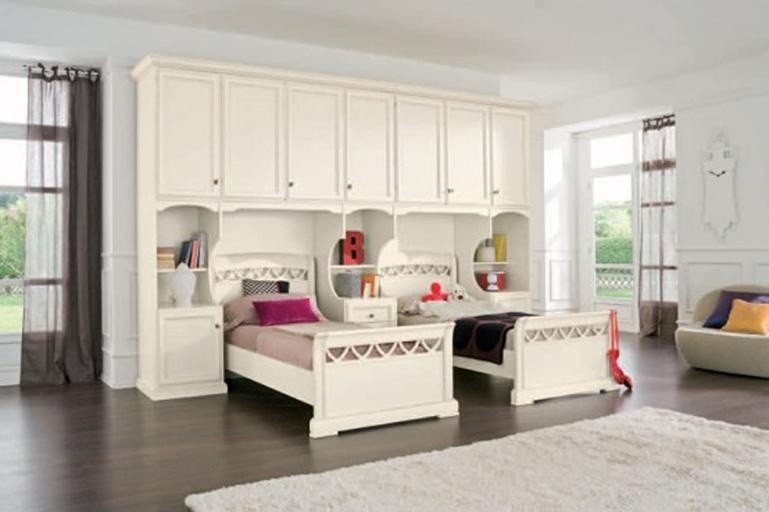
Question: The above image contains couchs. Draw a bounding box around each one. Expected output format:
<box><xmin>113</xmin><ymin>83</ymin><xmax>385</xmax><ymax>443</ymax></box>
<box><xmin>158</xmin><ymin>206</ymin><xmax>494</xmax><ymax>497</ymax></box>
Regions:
<box><xmin>674</xmin><ymin>282</ymin><xmax>769</xmax><ymax>380</ymax></box>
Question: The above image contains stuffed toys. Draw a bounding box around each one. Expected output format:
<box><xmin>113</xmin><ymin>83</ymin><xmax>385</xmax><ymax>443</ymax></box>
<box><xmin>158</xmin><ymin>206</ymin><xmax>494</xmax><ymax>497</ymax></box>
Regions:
<box><xmin>447</xmin><ymin>286</ymin><xmax>469</xmax><ymax>302</ymax></box>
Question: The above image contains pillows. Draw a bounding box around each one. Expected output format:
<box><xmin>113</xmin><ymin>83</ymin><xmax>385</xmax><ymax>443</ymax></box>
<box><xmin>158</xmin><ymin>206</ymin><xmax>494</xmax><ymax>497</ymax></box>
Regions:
<box><xmin>224</xmin><ymin>292</ymin><xmax>329</xmax><ymax>334</ymax></box>
<box><xmin>720</xmin><ymin>298</ymin><xmax>769</xmax><ymax>335</ymax></box>
<box><xmin>250</xmin><ymin>297</ymin><xmax>320</xmax><ymax>327</ymax></box>
<box><xmin>241</xmin><ymin>278</ymin><xmax>289</xmax><ymax>296</ymax></box>
<box><xmin>701</xmin><ymin>290</ymin><xmax>769</xmax><ymax>329</ymax></box>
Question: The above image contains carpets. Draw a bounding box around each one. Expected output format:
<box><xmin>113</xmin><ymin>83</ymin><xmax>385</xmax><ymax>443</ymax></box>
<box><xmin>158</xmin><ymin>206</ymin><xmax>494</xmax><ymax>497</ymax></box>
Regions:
<box><xmin>182</xmin><ymin>405</ymin><xmax>769</xmax><ymax>512</ymax></box>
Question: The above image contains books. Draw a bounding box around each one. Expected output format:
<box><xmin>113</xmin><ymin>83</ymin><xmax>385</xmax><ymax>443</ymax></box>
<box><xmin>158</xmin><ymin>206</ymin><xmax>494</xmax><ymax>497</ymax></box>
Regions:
<box><xmin>337</xmin><ymin>272</ymin><xmax>380</xmax><ymax>298</ymax></box>
<box><xmin>157</xmin><ymin>232</ymin><xmax>208</xmax><ymax>270</ymax></box>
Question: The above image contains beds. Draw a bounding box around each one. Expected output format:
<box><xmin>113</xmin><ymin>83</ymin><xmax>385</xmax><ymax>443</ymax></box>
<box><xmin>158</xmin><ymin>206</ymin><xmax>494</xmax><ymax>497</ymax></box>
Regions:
<box><xmin>376</xmin><ymin>236</ymin><xmax>633</xmax><ymax>407</ymax></box>
<box><xmin>212</xmin><ymin>250</ymin><xmax>460</xmax><ymax>440</ymax></box>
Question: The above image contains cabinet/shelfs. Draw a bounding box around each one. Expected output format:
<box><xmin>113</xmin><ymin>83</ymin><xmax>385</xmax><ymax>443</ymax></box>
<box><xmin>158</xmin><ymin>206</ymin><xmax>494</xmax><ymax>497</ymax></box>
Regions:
<box><xmin>160</xmin><ymin>67</ymin><xmax>285</xmax><ymax>200</ymax></box>
<box><xmin>284</xmin><ymin>81</ymin><xmax>392</xmax><ymax>202</ymax></box>
<box><xmin>487</xmin><ymin>107</ymin><xmax>530</xmax><ymax>202</ymax></box>
<box><xmin>396</xmin><ymin>96</ymin><xmax>487</xmax><ymax>201</ymax></box>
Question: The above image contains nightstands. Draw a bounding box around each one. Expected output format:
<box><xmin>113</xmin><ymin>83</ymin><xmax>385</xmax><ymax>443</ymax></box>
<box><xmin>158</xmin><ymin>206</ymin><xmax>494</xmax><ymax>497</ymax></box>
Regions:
<box><xmin>343</xmin><ymin>298</ymin><xmax>398</xmax><ymax>327</ymax></box>
<box><xmin>157</xmin><ymin>303</ymin><xmax>229</xmax><ymax>399</ymax></box>
<box><xmin>493</xmin><ymin>292</ymin><xmax>535</xmax><ymax>312</ymax></box>
